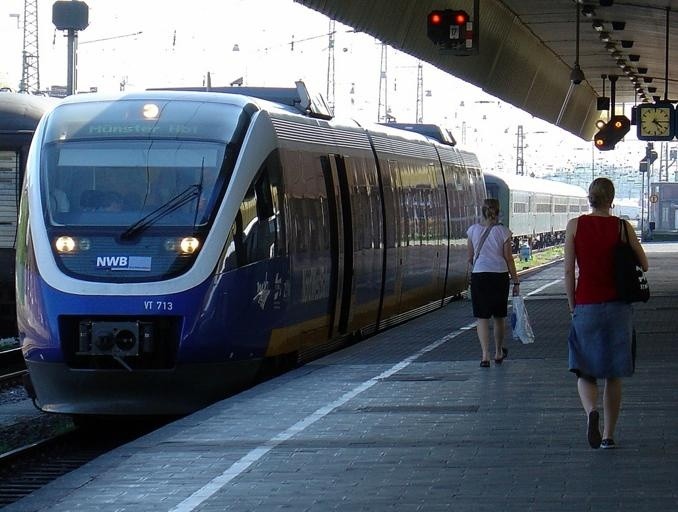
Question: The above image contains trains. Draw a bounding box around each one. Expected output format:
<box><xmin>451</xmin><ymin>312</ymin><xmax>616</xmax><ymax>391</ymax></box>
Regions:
<box><xmin>1</xmin><ymin>81</ymin><xmax>70</xmax><ymax>341</ymax></box>
<box><xmin>12</xmin><ymin>80</ymin><xmax>642</xmax><ymax>415</ymax></box>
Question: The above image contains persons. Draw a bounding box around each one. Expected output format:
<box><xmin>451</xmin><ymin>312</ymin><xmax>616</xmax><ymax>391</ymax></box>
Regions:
<box><xmin>467</xmin><ymin>198</ymin><xmax>520</xmax><ymax>367</ymax></box>
<box><xmin>564</xmin><ymin>177</ymin><xmax>650</xmax><ymax>451</ymax></box>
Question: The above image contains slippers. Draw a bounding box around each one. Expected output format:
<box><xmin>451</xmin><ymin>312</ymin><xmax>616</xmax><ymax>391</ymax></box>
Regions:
<box><xmin>495</xmin><ymin>347</ymin><xmax>508</xmax><ymax>364</ymax></box>
<box><xmin>480</xmin><ymin>360</ymin><xmax>490</xmax><ymax>367</ymax></box>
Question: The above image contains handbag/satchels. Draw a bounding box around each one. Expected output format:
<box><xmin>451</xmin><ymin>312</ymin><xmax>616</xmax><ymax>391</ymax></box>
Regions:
<box><xmin>611</xmin><ymin>219</ymin><xmax>650</xmax><ymax>304</ymax></box>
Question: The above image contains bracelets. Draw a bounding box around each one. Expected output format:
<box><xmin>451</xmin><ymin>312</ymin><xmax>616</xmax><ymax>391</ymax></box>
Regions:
<box><xmin>512</xmin><ymin>282</ymin><xmax>519</xmax><ymax>286</ymax></box>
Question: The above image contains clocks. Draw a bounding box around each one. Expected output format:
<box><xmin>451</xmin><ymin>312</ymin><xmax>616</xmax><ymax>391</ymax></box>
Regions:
<box><xmin>635</xmin><ymin>104</ymin><xmax>672</xmax><ymax>141</ymax></box>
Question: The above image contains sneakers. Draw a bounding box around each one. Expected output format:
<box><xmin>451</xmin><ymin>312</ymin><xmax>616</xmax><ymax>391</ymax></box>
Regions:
<box><xmin>586</xmin><ymin>408</ymin><xmax>602</xmax><ymax>449</ymax></box>
<box><xmin>600</xmin><ymin>439</ymin><xmax>615</xmax><ymax>449</ymax></box>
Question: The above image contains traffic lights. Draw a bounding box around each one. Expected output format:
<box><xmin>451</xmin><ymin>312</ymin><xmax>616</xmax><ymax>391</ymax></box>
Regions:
<box><xmin>594</xmin><ymin>116</ymin><xmax>630</xmax><ymax>152</ymax></box>
<box><xmin>426</xmin><ymin>10</ymin><xmax>469</xmax><ymax>42</ymax></box>
